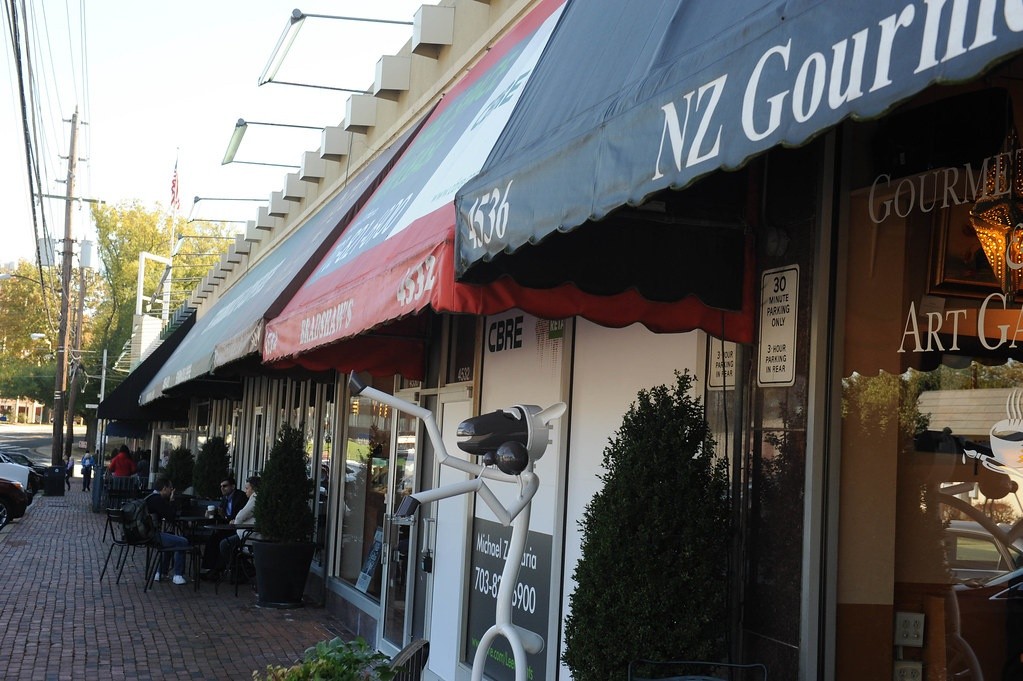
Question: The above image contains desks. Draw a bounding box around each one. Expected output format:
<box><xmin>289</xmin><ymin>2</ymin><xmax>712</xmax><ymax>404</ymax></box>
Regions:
<box><xmin>204</xmin><ymin>523</ymin><xmax>254</xmax><ymax>597</ymax></box>
<box><xmin>173</xmin><ymin>516</ymin><xmax>216</xmax><ymax>578</ymax></box>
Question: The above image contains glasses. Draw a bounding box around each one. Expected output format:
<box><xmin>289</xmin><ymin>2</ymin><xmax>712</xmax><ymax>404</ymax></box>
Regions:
<box><xmin>219</xmin><ymin>484</ymin><xmax>231</xmax><ymax>488</ymax></box>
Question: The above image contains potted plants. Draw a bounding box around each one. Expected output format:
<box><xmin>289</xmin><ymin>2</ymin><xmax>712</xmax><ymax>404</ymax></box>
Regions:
<box><xmin>251</xmin><ymin>421</ymin><xmax>318</xmax><ymax>607</ymax></box>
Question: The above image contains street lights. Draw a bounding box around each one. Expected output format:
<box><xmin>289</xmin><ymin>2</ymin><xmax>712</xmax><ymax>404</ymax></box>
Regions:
<box><xmin>31</xmin><ymin>332</ymin><xmax>69</xmax><ymax>466</ymax></box>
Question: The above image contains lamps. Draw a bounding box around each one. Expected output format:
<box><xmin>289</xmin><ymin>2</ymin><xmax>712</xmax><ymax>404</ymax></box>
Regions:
<box><xmin>258</xmin><ymin>8</ymin><xmax>413</xmax><ymax>96</ymax></box>
<box><xmin>112</xmin><ymin>264</ymin><xmax>213</xmax><ymax>372</ymax></box>
<box><xmin>187</xmin><ymin>196</ymin><xmax>269</xmax><ymax>223</ymax></box>
<box><xmin>222</xmin><ymin>118</ymin><xmax>324</xmax><ymax>169</ymax></box>
<box><xmin>171</xmin><ymin>234</ymin><xmax>235</xmax><ymax>257</ymax></box>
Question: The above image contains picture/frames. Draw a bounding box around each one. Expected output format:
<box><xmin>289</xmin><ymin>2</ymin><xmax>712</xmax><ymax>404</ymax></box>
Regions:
<box><xmin>925</xmin><ymin>202</ymin><xmax>1023</xmax><ymax>305</ymax></box>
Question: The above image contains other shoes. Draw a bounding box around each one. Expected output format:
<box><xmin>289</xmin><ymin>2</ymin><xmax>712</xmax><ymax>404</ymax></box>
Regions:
<box><xmin>200</xmin><ymin>569</ymin><xmax>221</xmax><ymax>582</ymax></box>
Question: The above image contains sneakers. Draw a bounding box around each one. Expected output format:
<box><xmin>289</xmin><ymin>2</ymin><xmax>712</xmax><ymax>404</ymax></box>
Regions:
<box><xmin>173</xmin><ymin>575</ymin><xmax>186</xmax><ymax>585</ymax></box>
<box><xmin>154</xmin><ymin>572</ymin><xmax>172</xmax><ymax>581</ymax></box>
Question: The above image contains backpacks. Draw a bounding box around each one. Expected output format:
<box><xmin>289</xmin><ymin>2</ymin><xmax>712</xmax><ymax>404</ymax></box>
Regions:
<box><xmin>118</xmin><ymin>493</ymin><xmax>161</xmax><ymax>544</ymax></box>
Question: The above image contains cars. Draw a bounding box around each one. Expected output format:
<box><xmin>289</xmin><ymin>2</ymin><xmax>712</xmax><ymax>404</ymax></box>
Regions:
<box><xmin>0</xmin><ymin>477</ymin><xmax>27</xmax><ymax>531</ymax></box>
<box><xmin>310</xmin><ymin>458</ymin><xmax>365</xmax><ymax>487</ymax></box>
<box><xmin>953</xmin><ymin>565</ymin><xmax>1023</xmax><ymax>664</ymax></box>
<box><xmin>946</xmin><ymin>519</ymin><xmax>1022</xmax><ymax>583</ymax></box>
<box><xmin>0</xmin><ymin>453</ymin><xmax>40</xmax><ymax>497</ymax></box>
<box><xmin>0</xmin><ymin>451</ymin><xmax>49</xmax><ymax>491</ymax></box>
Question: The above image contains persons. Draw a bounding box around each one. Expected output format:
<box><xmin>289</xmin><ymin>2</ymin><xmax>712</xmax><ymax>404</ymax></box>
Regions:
<box><xmin>108</xmin><ymin>444</ymin><xmax>152</xmax><ymax>479</ymax></box>
<box><xmin>62</xmin><ymin>455</ymin><xmax>74</xmax><ymax>490</ymax></box>
<box><xmin>81</xmin><ymin>450</ymin><xmax>95</xmax><ymax>491</ymax></box>
<box><xmin>197</xmin><ymin>479</ymin><xmax>248</xmax><ymax>583</ymax></box>
<box><xmin>142</xmin><ymin>478</ymin><xmax>188</xmax><ymax>584</ymax></box>
<box><xmin>219</xmin><ymin>476</ymin><xmax>261</xmax><ymax>592</ymax></box>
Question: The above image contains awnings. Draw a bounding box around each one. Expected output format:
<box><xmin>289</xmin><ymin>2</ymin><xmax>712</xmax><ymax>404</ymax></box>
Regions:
<box><xmin>95</xmin><ymin>313</ymin><xmax>196</xmax><ymax>421</ymax></box>
<box><xmin>455</xmin><ymin>0</ymin><xmax>1023</xmax><ymax>315</ymax></box>
<box><xmin>261</xmin><ymin>0</ymin><xmax>753</xmax><ymax>380</ymax></box>
<box><xmin>136</xmin><ymin>102</ymin><xmax>437</xmax><ymax>404</ymax></box>
<box><xmin>106</xmin><ymin>419</ymin><xmax>147</xmax><ymax>440</ymax></box>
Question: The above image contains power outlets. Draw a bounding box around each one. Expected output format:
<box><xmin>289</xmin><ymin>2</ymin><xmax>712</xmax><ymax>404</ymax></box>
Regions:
<box><xmin>892</xmin><ymin>660</ymin><xmax>922</xmax><ymax>681</ymax></box>
<box><xmin>894</xmin><ymin>611</ymin><xmax>924</xmax><ymax>647</ymax></box>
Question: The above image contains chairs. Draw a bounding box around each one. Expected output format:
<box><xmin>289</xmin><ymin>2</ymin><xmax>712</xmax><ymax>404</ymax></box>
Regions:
<box><xmin>628</xmin><ymin>659</ymin><xmax>767</xmax><ymax>681</ymax></box>
<box><xmin>98</xmin><ymin>475</ymin><xmax>256</xmax><ymax>597</ymax></box>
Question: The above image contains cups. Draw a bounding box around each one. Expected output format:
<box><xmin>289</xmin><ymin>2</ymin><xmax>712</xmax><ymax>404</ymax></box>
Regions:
<box><xmin>208</xmin><ymin>506</ymin><xmax>215</xmax><ymax>519</ymax></box>
